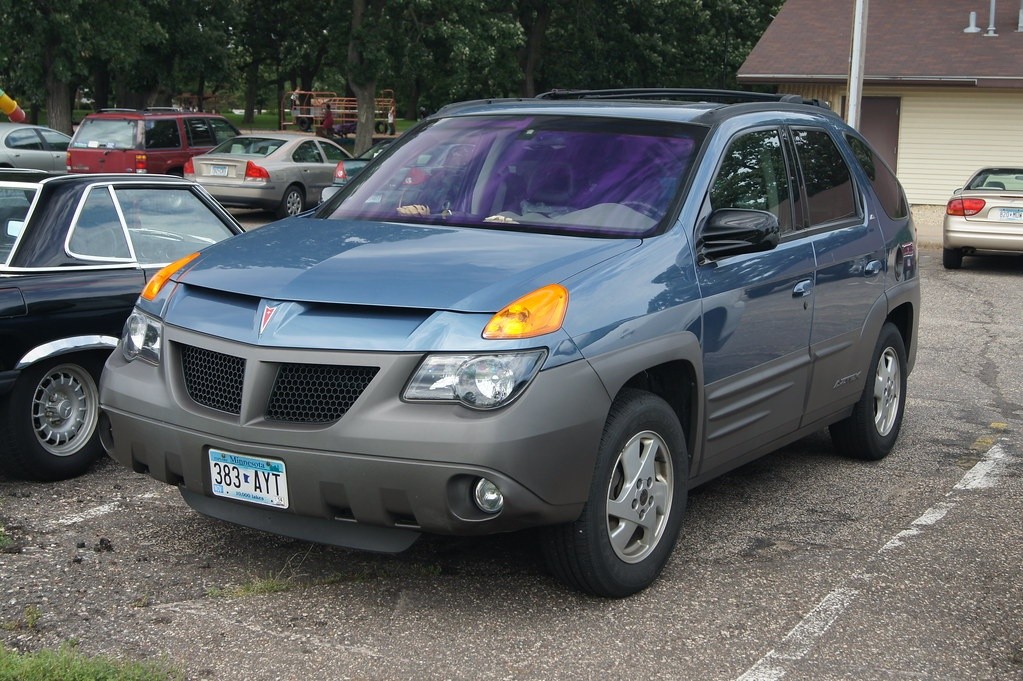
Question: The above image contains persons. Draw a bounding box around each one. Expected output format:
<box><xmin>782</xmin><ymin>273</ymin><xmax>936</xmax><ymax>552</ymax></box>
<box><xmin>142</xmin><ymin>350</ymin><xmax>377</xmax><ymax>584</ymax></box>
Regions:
<box><xmin>322</xmin><ymin>104</ymin><xmax>332</xmax><ymax>133</ymax></box>
<box><xmin>388</xmin><ymin>106</ymin><xmax>395</xmax><ymax>135</ymax></box>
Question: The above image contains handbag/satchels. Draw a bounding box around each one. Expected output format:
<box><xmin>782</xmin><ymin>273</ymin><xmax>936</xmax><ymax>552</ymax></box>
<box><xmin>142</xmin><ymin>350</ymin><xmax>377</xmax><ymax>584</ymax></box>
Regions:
<box><xmin>320</xmin><ymin>121</ymin><xmax>323</xmax><ymax>125</ymax></box>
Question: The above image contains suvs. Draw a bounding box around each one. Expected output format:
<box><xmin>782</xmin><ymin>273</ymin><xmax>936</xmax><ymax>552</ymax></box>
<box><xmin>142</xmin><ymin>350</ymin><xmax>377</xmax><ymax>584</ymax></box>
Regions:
<box><xmin>66</xmin><ymin>106</ymin><xmax>244</xmax><ymax>174</ymax></box>
<box><xmin>95</xmin><ymin>86</ymin><xmax>924</xmax><ymax>599</ymax></box>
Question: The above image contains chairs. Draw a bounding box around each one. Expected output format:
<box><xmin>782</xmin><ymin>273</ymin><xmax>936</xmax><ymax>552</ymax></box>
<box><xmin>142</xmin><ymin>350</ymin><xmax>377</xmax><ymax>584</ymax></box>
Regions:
<box><xmin>986</xmin><ymin>182</ymin><xmax>1006</xmax><ymax>191</ymax></box>
<box><xmin>231</xmin><ymin>144</ymin><xmax>246</xmax><ymax>153</ymax></box>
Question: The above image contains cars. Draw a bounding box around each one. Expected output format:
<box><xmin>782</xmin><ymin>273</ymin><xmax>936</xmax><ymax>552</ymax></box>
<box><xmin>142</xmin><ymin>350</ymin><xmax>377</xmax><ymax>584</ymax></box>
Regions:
<box><xmin>182</xmin><ymin>132</ymin><xmax>357</xmax><ymax>220</ymax></box>
<box><xmin>0</xmin><ymin>121</ymin><xmax>73</xmax><ymax>173</ymax></box>
<box><xmin>941</xmin><ymin>165</ymin><xmax>1023</xmax><ymax>270</ymax></box>
<box><xmin>0</xmin><ymin>168</ymin><xmax>247</xmax><ymax>483</ymax></box>
<box><xmin>319</xmin><ymin>136</ymin><xmax>477</xmax><ymax>216</ymax></box>
